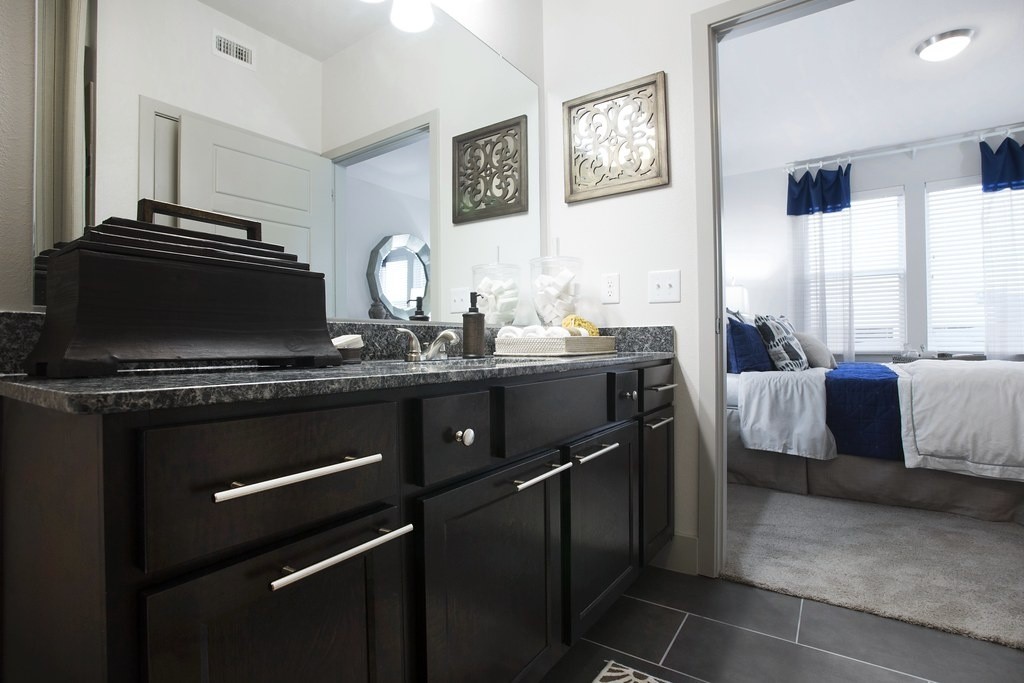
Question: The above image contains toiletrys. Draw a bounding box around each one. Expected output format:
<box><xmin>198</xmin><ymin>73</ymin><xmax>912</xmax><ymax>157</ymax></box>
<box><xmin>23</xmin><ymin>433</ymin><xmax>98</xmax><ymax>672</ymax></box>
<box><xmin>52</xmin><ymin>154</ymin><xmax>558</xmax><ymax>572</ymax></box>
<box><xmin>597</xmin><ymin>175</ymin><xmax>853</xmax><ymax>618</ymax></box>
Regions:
<box><xmin>463</xmin><ymin>291</ymin><xmax>487</xmax><ymax>359</ymax></box>
<box><xmin>405</xmin><ymin>296</ymin><xmax>431</xmax><ymax>321</ymax></box>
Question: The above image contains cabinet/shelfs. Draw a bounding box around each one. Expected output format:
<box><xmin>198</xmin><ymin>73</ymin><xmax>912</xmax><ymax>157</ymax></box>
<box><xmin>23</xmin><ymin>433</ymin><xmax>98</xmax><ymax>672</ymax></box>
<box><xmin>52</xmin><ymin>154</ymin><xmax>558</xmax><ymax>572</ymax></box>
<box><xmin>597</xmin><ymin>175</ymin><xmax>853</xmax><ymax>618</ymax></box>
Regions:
<box><xmin>0</xmin><ymin>355</ymin><xmax>677</xmax><ymax>683</ymax></box>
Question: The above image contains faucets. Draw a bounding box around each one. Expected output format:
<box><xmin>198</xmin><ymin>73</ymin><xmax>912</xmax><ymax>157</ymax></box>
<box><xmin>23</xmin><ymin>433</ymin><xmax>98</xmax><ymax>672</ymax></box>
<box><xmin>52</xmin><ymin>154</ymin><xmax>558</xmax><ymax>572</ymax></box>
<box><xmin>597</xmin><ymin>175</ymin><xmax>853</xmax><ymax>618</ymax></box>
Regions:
<box><xmin>394</xmin><ymin>327</ymin><xmax>459</xmax><ymax>362</ymax></box>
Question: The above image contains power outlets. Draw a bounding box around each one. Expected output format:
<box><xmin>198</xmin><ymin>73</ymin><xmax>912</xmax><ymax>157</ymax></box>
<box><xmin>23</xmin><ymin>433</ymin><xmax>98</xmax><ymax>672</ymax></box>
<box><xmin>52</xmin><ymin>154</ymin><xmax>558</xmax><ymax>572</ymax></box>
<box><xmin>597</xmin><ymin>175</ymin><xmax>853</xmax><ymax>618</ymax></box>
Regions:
<box><xmin>600</xmin><ymin>271</ymin><xmax>620</xmax><ymax>303</ymax></box>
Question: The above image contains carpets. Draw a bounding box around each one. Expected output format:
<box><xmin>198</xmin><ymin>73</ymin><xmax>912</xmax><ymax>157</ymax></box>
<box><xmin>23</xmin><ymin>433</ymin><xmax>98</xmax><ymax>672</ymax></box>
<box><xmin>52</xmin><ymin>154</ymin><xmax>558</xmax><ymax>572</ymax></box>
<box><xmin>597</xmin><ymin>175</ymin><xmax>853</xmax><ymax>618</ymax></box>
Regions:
<box><xmin>719</xmin><ymin>479</ymin><xmax>1024</xmax><ymax>653</ymax></box>
<box><xmin>591</xmin><ymin>659</ymin><xmax>672</xmax><ymax>683</ymax></box>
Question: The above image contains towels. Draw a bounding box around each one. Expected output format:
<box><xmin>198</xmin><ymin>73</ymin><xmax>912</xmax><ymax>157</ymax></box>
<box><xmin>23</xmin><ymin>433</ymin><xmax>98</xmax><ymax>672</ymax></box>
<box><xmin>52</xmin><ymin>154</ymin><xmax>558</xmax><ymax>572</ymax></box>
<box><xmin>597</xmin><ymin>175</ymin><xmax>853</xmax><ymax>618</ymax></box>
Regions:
<box><xmin>496</xmin><ymin>325</ymin><xmax>589</xmax><ymax>338</ymax></box>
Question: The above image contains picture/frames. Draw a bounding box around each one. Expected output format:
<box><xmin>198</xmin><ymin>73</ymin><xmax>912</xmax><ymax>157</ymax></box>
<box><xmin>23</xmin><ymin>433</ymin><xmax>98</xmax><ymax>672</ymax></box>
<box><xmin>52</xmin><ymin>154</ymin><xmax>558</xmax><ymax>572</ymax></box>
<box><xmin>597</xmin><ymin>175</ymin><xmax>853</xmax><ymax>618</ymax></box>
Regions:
<box><xmin>562</xmin><ymin>70</ymin><xmax>671</xmax><ymax>205</ymax></box>
<box><xmin>451</xmin><ymin>113</ymin><xmax>528</xmax><ymax>224</ymax></box>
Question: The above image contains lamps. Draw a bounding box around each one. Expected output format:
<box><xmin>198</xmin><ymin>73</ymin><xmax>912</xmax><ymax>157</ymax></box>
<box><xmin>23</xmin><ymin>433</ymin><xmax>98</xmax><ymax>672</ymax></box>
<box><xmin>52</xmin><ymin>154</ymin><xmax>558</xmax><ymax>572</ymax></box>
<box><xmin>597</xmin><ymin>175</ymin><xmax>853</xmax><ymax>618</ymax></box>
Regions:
<box><xmin>914</xmin><ymin>28</ymin><xmax>976</xmax><ymax>64</ymax></box>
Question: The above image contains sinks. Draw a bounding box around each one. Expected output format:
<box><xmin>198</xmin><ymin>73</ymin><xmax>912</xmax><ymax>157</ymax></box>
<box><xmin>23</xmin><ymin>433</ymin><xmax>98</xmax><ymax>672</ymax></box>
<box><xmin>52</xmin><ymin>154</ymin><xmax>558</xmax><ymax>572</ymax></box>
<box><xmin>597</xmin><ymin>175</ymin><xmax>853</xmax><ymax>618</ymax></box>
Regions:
<box><xmin>387</xmin><ymin>356</ymin><xmax>561</xmax><ymax>370</ymax></box>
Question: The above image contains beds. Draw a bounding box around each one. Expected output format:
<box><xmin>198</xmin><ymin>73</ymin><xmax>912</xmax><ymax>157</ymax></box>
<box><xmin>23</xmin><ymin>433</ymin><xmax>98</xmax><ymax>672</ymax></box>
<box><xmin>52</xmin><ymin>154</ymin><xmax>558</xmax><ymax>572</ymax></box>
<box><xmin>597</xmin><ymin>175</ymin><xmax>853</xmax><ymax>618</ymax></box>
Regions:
<box><xmin>722</xmin><ymin>284</ymin><xmax>1024</xmax><ymax>525</ymax></box>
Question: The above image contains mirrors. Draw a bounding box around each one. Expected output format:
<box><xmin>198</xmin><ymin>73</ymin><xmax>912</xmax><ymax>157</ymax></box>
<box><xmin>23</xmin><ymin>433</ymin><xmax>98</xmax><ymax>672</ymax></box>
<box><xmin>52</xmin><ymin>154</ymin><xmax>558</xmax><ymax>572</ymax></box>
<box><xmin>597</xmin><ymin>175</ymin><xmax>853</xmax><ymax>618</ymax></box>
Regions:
<box><xmin>91</xmin><ymin>0</ymin><xmax>542</xmax><ymax>329</ymax></box>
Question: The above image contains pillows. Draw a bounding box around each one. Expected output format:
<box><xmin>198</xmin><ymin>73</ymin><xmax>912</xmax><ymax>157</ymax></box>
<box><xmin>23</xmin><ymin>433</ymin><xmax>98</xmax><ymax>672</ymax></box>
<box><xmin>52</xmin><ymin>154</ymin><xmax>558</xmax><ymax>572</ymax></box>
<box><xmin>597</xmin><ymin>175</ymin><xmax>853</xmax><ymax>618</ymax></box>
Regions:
<box><xmin>726</xmin><ymin>305</ymin><xmax>780</xmax><ymax>375</ymax></box>
<box><xmin>753</xmin><ymin>312</ymin><xmax>808</xmax><ymax>372</ymax></box>
<box><xmin>776</xmin><ymin>313</ymin><xmax>796</xmax><ymax>333</ymax></box>
<box><xmin>792</xmin><ymin>331</ymin><xmax>840</xmax><ymax>370</ymax></box>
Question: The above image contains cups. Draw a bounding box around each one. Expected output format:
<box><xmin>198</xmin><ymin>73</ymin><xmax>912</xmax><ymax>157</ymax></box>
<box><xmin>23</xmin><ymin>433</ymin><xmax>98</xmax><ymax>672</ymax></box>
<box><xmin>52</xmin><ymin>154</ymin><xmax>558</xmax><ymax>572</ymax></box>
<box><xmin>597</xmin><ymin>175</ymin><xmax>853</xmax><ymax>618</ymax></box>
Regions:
<box><xmin>529</xmin><ymin>256</ymin><xmax>584</xmax><ymax>328</ymax></box>
<box><xmin>471</xmin><ymin>263</ymin><xmax>526</xmax><ymax>327</ymax></box>
